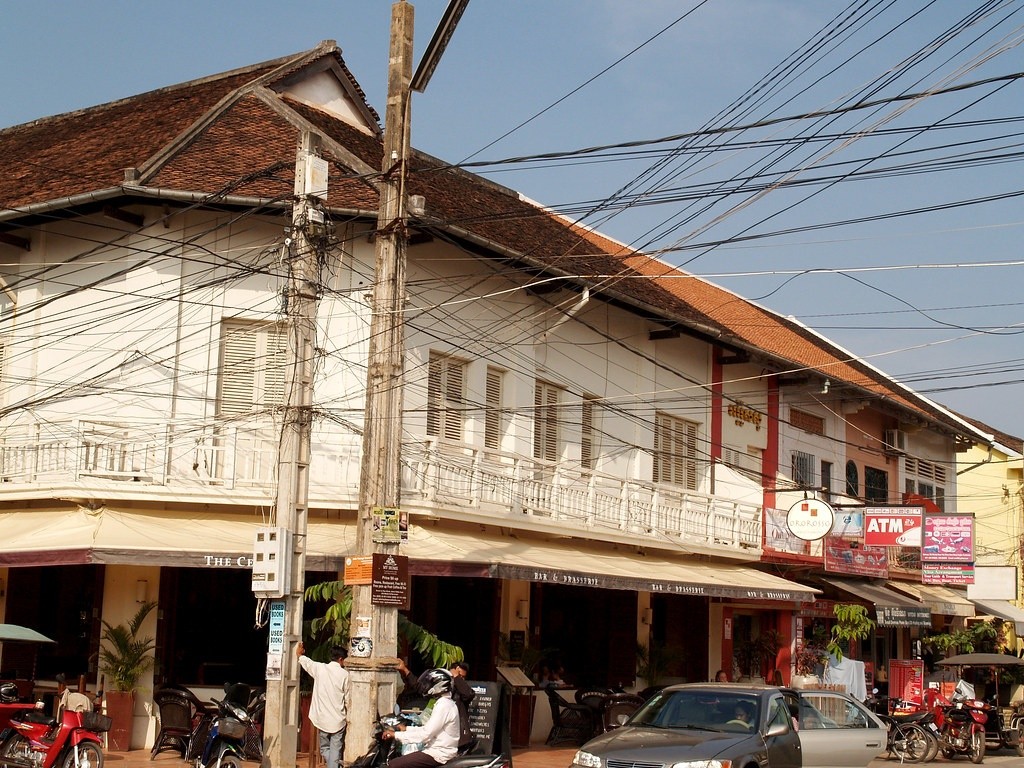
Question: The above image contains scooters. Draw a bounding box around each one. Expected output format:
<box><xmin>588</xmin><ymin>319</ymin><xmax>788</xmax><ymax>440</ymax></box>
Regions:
<box><xmin>348</xmin><ymin>702</ymin><xmax>513</xmax><ymax>768</ymax></box>
<box><xmin>0</xmin><ymin>690</ymin><xmax>113</xmax><ymax>768</ymax></box>
<box><xmin>190</xmin><ymin>682</ymin><xmax>254</xmax><ymax>768</ymax></box>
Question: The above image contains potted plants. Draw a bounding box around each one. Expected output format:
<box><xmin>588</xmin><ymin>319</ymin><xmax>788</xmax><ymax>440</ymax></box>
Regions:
<box><xmin>803</xmin><ymin>604</ymin><xmax>876</xmax><ymax>729</ymax></box>
<box><xmin>493</xmin><ymin>630</ymin><xmax>541</xmax><ymax>747</ymax></box>
<box><xmin>86</xmin><ymin>600</ymin><xmax>162</xmax><ymax>752</ymax></box>
<box><xmin>629</xmin><ymin>640</ymin><xmax>686</xmax><ymax>719</ymax></box>
<box><xmin>739</xmin><ymin>630</ymin><xmax>784</xmax><ymax>685</ymax></box>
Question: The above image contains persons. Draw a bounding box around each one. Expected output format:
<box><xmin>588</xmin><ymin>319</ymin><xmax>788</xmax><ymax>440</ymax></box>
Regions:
<box><xmin>296</xmin><ymin>642</ymin><xmax>475</xmax><ymax>768</ymax></box>
<box><xmin>734</xmin><ymin>701</ymin><xmax>754</xmax><ymax>728</ymax></box>
<box><xmin>716</xmin><ymin>670</ymin><xmax>728</xmax><ymax>682</ymax></box>
<box><xmin>533</xmin><ymin>663</ymin><xmax>586</xmax><ymax>689</ymax></box>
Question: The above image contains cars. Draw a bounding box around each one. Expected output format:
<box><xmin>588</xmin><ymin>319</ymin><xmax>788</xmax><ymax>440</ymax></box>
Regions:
<box><xmin>565</xmin><ymin>681</ymin><xmax>889</xmax><ymax>768</ymax></box>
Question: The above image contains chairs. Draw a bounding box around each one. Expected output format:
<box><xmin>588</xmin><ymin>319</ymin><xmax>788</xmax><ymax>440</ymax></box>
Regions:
<box><xmin>544</xmin><ymin>685</ymin><xmax>677</xmax><ymax>748</ymax></box>
<box><xmin>150</xmin><ymin>680</ymin><xmax>266</xmax><ymax>764</ymax></box>
<box><xmin>682</xmin><ymin>702</ymin><xmax>708</xmax><ymax>721</ymax></box>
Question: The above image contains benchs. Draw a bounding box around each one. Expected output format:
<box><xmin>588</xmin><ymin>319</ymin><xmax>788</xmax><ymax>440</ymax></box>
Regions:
<box><xmin>974</xmin><ymin>684</ymin><xmax>1024</xmax><ymax>727</ymax></box>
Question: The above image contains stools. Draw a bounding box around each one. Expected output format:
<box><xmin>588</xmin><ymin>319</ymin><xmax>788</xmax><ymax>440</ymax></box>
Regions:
<box><xmin>56</xmin><ymin>672</ymin><xmax>104</xmax><ymax>717</ymax></box>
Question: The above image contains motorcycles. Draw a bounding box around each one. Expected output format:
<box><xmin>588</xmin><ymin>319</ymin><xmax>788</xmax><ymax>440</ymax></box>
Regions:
<box><xmin>844</xmin><ymin>654</ymin><xmax>1024</xmax><ymax>763</ymax></box>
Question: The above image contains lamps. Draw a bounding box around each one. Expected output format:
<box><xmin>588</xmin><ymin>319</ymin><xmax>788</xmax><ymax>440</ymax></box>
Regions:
<box><xmin>516</xmin><ymin>599</ymin><xmax>528</xmax><ymax>619</ymax></box>
<box><xmin>137</xmin><ymin>579</ymin><xmax>148</xmax><ymax>603</ymax></box>
<box><xmin>642</xmin><ymin>608</ymin><xmax>652</xmax><ymax>625</ymax></box>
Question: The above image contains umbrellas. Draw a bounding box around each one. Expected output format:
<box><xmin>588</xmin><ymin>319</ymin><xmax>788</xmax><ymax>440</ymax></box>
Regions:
<box><xmin>935</xmin><ymin>653</ymin><xmax>1024</xmax><ymax>711</ymax></box>
<box><xmin>0</xmin><ymin>624</ymin><xmax>55</xmax><ymax>644</ymax></box>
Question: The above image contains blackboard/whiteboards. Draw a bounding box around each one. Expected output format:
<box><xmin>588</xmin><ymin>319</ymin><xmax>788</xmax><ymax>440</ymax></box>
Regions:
<box><xmin>464</xmin><ymin>681</ymin><xmax>504</xmax><ymax>759</ymax></box>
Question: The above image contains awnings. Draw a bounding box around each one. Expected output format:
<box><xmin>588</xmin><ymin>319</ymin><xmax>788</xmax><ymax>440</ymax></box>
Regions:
<box><xmin>886</xmin><ymin>580</ymin><xmax>976</xmax><ymax>617</ymax></box>
<box><xmin>821</xmin><ymin>576</ymin><xmax>932</xmax><ymax>626</ymax></box>
<box><xmin>0</xmin><ymin>509</ymin><xmax>823</xmax><ymax>602</ymax></box>
<box><xmin>974</xmin><ymin>599</ymin><xmax>1024</xmax><ymax>637</ymax></box>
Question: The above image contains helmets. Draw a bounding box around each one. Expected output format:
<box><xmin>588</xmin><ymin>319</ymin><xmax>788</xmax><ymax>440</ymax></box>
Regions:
<box><xmin>419</xmin><ymin>667</ymin><xmax>453</xmax><ymax>697</ymax></box>
<box><xmin>0</xmin><ymin>683</ymin><xmax>19</xmax><ymax>703</ymax></box>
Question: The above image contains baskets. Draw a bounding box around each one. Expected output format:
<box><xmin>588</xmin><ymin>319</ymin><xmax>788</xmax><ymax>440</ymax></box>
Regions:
<box><xmin>84</xmin><ymin>711</ymin><xmax>113</xmax><ymax>732</ymax></box>
<box><xmin>218</xmin><ymin>717</ymin><xmax>248</xmax><ymax>738</ymax></box>
